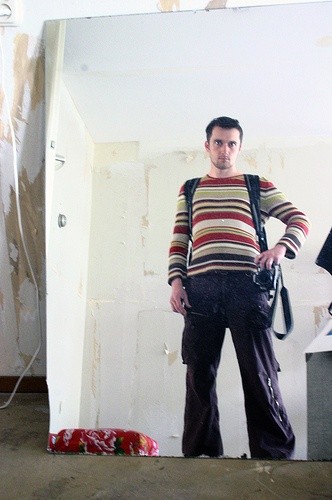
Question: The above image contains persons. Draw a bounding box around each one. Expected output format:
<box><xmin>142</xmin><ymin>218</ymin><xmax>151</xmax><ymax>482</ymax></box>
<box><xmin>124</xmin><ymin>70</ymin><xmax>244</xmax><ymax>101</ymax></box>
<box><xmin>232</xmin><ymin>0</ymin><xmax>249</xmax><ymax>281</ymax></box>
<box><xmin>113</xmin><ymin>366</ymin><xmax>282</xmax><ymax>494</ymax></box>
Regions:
<box><xmin>168</xmin><ymin>117</ymin><xmax>310</xmax><ymax>460</ymax></box>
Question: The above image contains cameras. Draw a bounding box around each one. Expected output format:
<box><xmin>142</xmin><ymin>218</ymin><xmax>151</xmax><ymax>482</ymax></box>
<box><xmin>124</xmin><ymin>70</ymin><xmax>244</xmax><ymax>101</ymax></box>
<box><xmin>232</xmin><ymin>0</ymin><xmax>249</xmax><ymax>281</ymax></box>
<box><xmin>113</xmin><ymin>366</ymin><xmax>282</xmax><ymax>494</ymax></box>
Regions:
<box><xmin>253</xmin><ymin>263</ymin><xmax>279</xmax><ymax>290</ymax></box>
